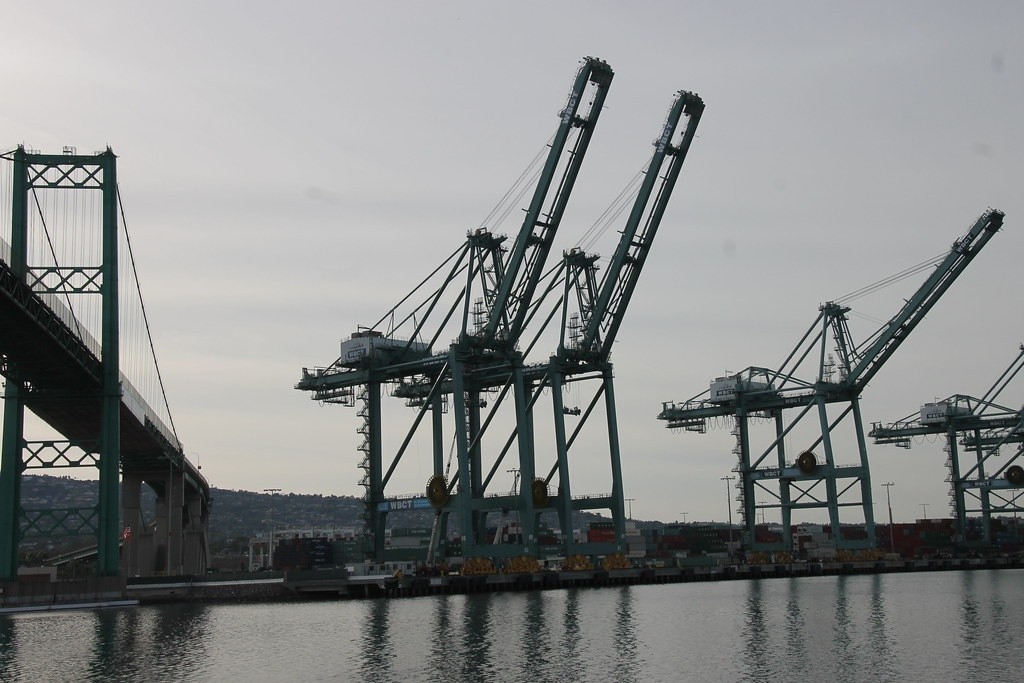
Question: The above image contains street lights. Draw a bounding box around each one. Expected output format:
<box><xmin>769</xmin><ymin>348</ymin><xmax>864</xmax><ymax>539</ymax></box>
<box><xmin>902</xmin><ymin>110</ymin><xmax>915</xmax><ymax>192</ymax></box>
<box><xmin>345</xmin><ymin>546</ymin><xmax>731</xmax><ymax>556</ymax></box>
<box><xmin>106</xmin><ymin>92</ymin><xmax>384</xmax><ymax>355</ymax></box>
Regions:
<box><xmin>263</xmin><ymin>489</ymin><xmax>282</xmax><ymax>566</ymax></box>
<box><xmin>506</xmin><ymin>468</ymin><xmax>522</xmax><ymax>543</ymax></box>
<box><xmin>882</xmin><ymin>481</ymin><xmax>894</xmax><ymax>554</ymax></box>
<box><xmin>624</xmin><ymin>498</ymin><xmax>636</xmax><ymax>520</ymax></box>
<box><xmin>721</xmin><ymin>475</ymin><xmax>736</xmax><ymax>552</ymax></box>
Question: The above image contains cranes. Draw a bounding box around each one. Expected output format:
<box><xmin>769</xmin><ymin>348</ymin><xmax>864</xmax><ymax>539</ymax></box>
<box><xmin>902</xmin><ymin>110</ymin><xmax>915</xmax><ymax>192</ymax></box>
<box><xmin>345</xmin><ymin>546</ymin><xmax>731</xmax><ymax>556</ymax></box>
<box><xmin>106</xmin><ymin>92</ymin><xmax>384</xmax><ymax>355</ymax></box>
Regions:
<box><xmin>657</xmin><ymin>205</ymin><xmax>1010</xmax><ymax>420</ymax></box>
<box><xmin>391</xmin><ymin>89</ymin><xmax>705</xmax><ymax>397</ymax></box>
<box><xmin>294</xmin><ymin>54</ymin><xmax>615</xmax><ymax>392</ymax></box>
<box><xmin>866</xmin><ymin>344</ymin><xmax>1024</xmax><ymax>438</ymax></box>
